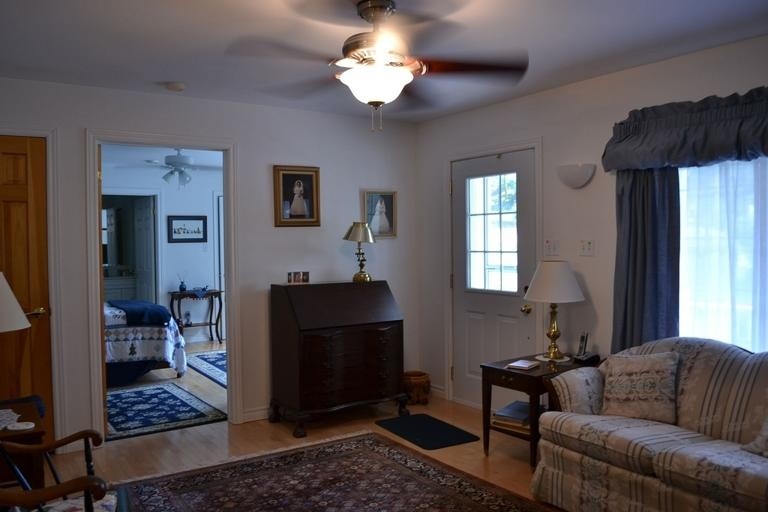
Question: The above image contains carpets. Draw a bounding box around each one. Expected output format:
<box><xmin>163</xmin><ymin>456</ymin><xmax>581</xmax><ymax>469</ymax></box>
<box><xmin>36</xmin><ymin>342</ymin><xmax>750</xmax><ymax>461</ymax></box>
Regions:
<box><xmin>106</xmin><ymin>382</ymin><xmax>227</xmax><ymax>442</ymax></box>
<box><xmin>185</xmin><ymin>350</ymin><xmax>227</xmax><ymax>389</ymax></box>
<box><xmin>107</xmin><ymin>429</ymin><xmax>551</xmax><ymax>511</ymax></box>
<box><xmin>376</xmin><ymin>413</ymin><xmax>480</xmax><ymax>451</ymax></box>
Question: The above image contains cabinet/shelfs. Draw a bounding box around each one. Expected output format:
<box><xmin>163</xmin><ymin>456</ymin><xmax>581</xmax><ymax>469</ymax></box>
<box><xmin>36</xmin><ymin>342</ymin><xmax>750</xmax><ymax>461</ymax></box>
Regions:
<box><xmin>268</xmin><ymin>281</ymin><xmax>410</xmax><ymax>439</ymax></box>
<box><xmin>104</xmin><ymin>277</ymin><xmax>136</xmax><ymax>300</ymax></box>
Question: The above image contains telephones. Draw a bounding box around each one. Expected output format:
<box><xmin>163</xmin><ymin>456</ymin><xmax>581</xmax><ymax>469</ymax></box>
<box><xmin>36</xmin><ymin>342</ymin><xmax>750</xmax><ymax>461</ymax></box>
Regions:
<box><xmin>574</xmin><ymin>330</ymin><xmax>600</xmax><ymax>365</ymax></box>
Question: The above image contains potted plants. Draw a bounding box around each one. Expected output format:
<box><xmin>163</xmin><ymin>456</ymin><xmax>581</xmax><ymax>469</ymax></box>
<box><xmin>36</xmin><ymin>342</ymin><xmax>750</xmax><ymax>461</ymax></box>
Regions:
<box><xmin>177</xmin><ymin>273</ymin><xmax>186</xmax><ymax>291</ymax></box>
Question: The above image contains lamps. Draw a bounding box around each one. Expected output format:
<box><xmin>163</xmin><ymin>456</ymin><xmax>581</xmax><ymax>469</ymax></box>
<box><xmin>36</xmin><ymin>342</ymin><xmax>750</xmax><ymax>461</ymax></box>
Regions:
<box><xmin>1</xmin><ymin>272</ymin><xmax>31</xmax><ymax>335</ymax></box>
<box><xmin>343</xmin><ymin>222</ymin><xmax>377</xmax><ymax>282</ymax></box>
<box><xmin>334</xmin><ymin>61</ymin><xmax>413</xmax><ymax>111</ymax></box>
<box><xmin>522</xmin><ymin>260</ymin><xmax>585</xmax><ymax>363</ymax></box>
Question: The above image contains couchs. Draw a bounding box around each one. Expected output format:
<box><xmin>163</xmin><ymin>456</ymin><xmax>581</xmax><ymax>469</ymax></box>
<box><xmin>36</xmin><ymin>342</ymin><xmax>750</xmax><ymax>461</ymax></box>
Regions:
<box><xmin>533</xmin><ymin>336</ymin><xmax>768</xmax><ymax>512</ymax></box>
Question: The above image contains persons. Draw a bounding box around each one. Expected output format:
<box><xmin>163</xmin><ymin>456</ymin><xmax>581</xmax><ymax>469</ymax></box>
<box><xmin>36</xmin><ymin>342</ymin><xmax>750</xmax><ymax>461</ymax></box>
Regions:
<box><xmin>368</xmin><ymin>199</ymin><xmax>391</xmax><ymax>235</ymax></box>
<box><xmin>289</xmin><ymin>179</ymin><xmax>307</xmax><ymax>218</ymax></box>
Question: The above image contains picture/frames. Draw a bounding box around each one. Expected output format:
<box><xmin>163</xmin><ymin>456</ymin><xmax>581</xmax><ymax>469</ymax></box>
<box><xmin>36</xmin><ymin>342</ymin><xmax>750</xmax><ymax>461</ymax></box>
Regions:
<box><xmin>168</xmin><ymin>216</ymin><xmax>208</xmax><ymax>243</ymax></box>
<box><xmin>273</xmin><ymin>165</ymin><xmax>320</xmax><ymax>227</ymax></box>
<box><xmin>359</xmin><ymin>189</ymin><xmax>396</xmax><ymax>239</ymax></box>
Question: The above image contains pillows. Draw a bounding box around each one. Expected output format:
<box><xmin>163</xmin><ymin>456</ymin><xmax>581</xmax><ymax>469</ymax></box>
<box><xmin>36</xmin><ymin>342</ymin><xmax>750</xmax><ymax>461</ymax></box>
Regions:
<box><xmin>602</xmin><ymin>351</ymin><xmax>680</xmax><ymax>421</ymax></box>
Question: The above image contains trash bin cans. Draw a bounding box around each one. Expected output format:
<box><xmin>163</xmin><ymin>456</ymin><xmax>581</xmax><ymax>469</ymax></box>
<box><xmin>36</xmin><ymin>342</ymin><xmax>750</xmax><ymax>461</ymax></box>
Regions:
<box><xmin>404</xmin><ymin>371</ymin><xmax>430</xmax><ymax>405</ymax></box>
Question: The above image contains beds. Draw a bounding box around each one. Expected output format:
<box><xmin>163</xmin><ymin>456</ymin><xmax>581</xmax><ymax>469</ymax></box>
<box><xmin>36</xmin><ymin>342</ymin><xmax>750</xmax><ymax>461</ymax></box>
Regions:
<box><xmin>104</xmin><ymin>300</ymin><xmax>174</xmax><ymax>380</ymax></box>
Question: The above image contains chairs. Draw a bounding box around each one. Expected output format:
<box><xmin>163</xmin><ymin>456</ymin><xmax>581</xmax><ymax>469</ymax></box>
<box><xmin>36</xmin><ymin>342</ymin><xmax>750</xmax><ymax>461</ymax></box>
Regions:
<box><xmin>0</xmin><ymin>428</ymin><xmax>107</xmax><ymax>512</ymax></box>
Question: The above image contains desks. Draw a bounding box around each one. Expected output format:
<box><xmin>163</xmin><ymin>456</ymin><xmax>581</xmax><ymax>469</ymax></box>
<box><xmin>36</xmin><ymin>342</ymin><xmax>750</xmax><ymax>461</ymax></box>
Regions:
<box><xmin>168</xmin><ymin>290</ymin><xmax>224</xmax><ymax>344</ymax></box>
<box><xmin>1</xmin><ymin>402</ymin><xmax>45</xmax><ymax>489</ymax></box>
<box><xmin>479</xmin><ymin>351</ymin><xmax>599</xmax><ymax>472</ymax></box>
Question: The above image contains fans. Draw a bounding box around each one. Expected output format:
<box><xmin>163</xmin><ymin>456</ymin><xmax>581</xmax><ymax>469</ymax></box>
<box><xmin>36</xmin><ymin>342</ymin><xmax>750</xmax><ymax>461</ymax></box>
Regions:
<box><xmin>224</xmin><ymin>1</ymin><xmax>529</xmax><ymax>100</ymax></box>
<box><xmin>145</xmin><ymin>149</ymin><xmax>223</xmax><ymax>184</ymax></box>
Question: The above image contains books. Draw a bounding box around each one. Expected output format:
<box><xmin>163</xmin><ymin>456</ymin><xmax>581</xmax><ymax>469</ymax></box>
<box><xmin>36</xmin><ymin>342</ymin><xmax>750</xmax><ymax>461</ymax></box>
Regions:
<box><xmin>492</xmin><ymin>400</ymin><xmax>546</xmax><ymax>435</ymax></box>
<box><xmin>506</xmin><ymin>359</ymin><xmax>540</xmax><ymax>370</ymax></box>
